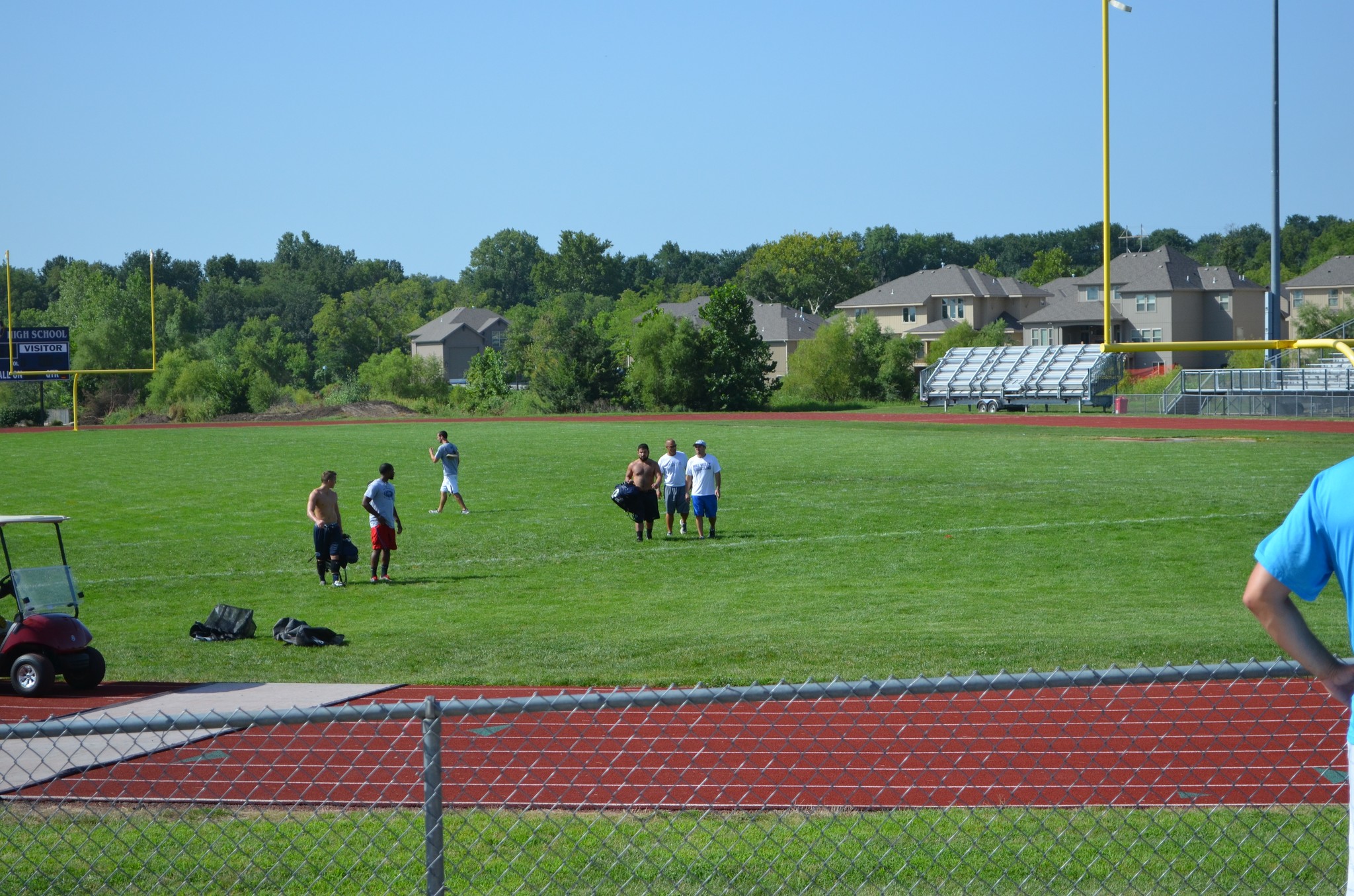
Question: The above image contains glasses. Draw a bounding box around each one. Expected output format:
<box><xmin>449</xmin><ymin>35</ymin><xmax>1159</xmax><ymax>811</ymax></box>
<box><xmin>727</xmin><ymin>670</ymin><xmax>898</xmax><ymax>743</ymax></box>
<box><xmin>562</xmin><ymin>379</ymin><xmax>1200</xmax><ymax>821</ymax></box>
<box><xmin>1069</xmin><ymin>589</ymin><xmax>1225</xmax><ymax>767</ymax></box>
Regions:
<box><xmin>668</xmin><ymin>444</ymin><xmax>677</xmax><ymax>448</ymax></box>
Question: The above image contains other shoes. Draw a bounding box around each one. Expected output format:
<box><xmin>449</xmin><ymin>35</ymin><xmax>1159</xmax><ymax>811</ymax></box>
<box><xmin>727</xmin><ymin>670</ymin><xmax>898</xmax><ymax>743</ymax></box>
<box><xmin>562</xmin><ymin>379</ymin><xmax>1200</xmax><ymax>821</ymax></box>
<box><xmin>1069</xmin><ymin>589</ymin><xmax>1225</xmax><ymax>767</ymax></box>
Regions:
<box><xmin>709</xmin><ymin>525</ymin><xmax>715</xmax><ymax>539</ymax></box>
<box><xmin>646</xmin><ymin>533</ymin><xmax>652</xmax><ymax>539</ymax></box>
<box><xmin>700</xmin><ymin>536</ymin><xmax>705</xmax><ymax>539</ymax></box>
<box><xmin>637</xmin><ymin>536</ymin><xmax>644</xmax><ymax>541</ymax></box>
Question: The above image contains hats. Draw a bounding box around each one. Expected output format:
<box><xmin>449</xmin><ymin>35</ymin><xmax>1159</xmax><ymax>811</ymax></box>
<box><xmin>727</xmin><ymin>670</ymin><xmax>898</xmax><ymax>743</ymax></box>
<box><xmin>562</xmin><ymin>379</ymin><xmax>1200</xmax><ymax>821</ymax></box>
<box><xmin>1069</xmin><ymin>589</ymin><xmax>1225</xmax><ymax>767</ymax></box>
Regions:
<box><xmin>445</xmin><ymin>453</ymin><xmax>458</xmax><ymax>460</ymax></box>
<box><xmin>693</xmin><ymin>440</ymin><xmax>706</xmax><ymax>448</ymax></box>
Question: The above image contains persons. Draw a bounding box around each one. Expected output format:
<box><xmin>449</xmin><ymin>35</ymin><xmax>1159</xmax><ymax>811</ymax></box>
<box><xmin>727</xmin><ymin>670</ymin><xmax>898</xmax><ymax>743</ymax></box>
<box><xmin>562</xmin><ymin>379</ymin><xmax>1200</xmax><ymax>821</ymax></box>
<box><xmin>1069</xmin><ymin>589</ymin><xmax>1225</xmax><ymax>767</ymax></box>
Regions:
<box><xmin>625</xmin><ymin>444</ymin><xmax>663</xmax><ymax>542</ymax></box>
<box><xmin>428</xmin><ymin>430</ymin><xmax>470</xmax><ymax>514</ymax></box>
<box><xmin>361</xmin><ymin>463</ymin><xmax>403</xmax><ymax>582</ymax></box>
<box><xmin>1243</xmin><ymin>456</ymin><xmax>1354</xmax><ymax>896</ymax></box>
<box><xmin>684</xmin><ymin>440</ymin><xmax>721</xmax><ymax>540</ymax></box>
<box><xmin>655</xmin><ymin>438</ymin><xmax>692</xmax><ymax>536</ymax></box>
<box><xmin>307</xmin><ymin>470</ymin><xmax>343</xmax><ymax>587</ymax></box>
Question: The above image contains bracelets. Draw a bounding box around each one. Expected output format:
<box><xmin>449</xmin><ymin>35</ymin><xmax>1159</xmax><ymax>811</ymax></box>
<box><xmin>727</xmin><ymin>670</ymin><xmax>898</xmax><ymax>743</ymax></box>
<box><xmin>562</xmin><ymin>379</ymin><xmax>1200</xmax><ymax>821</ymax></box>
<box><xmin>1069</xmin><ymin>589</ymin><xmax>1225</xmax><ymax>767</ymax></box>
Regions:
<box><xmin>716</xmin><ymin>488</ymin><xmax>720</xmax><ymax>491</ymax></box>
<box><xmin>685</xmin><ymin>493</ymin><xmax>689</xmax><ymax>495</ymax></box>
<box><xmin>378</xmin><ymin>515</ymin><xmax>381</xmax><ymax>519</ymax></box>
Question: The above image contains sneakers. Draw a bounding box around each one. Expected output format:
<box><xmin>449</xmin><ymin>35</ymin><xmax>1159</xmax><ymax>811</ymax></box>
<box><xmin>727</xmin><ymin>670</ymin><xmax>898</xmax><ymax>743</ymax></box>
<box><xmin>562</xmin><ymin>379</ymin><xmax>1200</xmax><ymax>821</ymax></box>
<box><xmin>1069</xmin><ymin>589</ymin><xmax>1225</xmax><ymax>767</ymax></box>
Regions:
<box><xmin>333</xmin><ymin>580</ymin><xmax>343</xmax><ymax>587</ymax></box>
<box><xmin>379</xmin><ymin>575</ymin><xmax>392</xmax><ymax>581</ymax></box>
<box><xmin>667</xmin><ymin>532</ymin><xmax>673</xmax><ymax>535</ymax></box>
<box><xmin>320</xmin><ymin>580</ymin><xmax>326</xmax><ymax>585</ymax></box>
<box><xmin>370</xmin><ymin>576</ymin><xmax>378</xmax><ymax>582</ymax></box>
<box><xmin>429</xmin><ymin>508</ymin><xmax>438</xmax><ymax>514</ymax></box>
<box><xmin>462</xmin><ymin>509</ymin><xmax>470</xmax><ymax>514</ymax></box>
<box><xmin>680</xmin><ymin>519</ymin><xmax>687</xmax><ymax>534</ymax></box>
